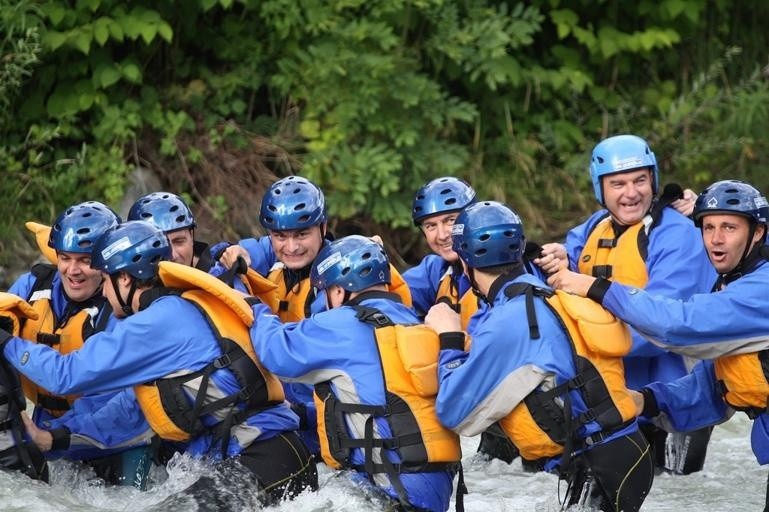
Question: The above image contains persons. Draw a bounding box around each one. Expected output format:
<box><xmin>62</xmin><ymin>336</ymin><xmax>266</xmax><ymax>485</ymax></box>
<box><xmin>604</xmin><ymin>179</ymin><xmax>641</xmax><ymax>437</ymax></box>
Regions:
<box><xmin>535</xmin><ymin>133</ymin><xmax>768</xmax><ymax>473</ymax></box>
<box><xmin>371</xmin><ymin>175</ymin><xmax>652</xmax><ymax>512</ymax></box>
<box><xmin>0</xmin><ymin>178</ymin><xmax>461</xmax><ymax>512</ymax></box>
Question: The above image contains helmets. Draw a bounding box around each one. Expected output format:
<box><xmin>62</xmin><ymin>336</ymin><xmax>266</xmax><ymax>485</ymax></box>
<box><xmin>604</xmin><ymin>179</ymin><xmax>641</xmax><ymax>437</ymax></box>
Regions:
<box><xmin>590</xmin><ymin>135</ymin><xmax>662</xmax><ymax>206</ymax></box>
<box><xmin>308</xmin><ymin>234</ymin><xmax>391</xmax><ymax>290</ymax></box>
<box><xmin>451</xmin><ymin>199</ymin><xmax>525</xmax><ymax>270</ymax></box>
<box><xmin>89</xmin><ymin>220</ymin><xmax>173</xmax><ymax>288</ymax></box>
<box><xmin>128</xmin><ymin>190</ymin><xmax>198</xmax><ymax>233</ymax></box>
<box><xmin>413</xmin><ymin>175</ymin><xmax>477</xmax><ymax>223</ymax></box>
<box><xmin>47</xmin><ymin>199</ymin><xmax>121</xmax><ymax>254</ymax></box>
<box><xmin>260</xmin><ymin>175</ymin><xmax>327</xmax><ymax>230</ymax></box>
<box><xmin>693</xmin><ymin>179</ymin><xmax>765</xmax><ymax>234</ymax></box>
